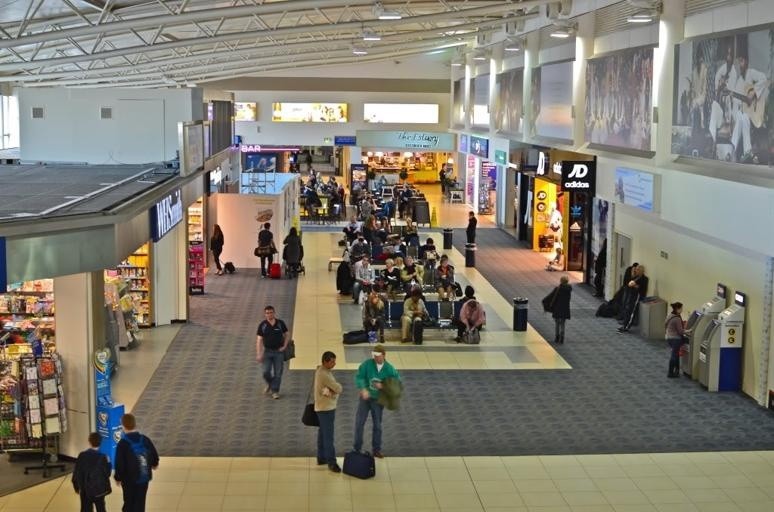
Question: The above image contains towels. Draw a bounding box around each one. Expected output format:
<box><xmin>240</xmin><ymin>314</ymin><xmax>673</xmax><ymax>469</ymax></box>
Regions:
<box><xmin>513</xmin><ymin>297</ymin><xmax>529</xmax><ymax>331</ymax></box>
<box><xmin>444</xmin><ymin>228</ymin><xmax>454</xmax><ymax>248</ymax></box>
<box><xmin>464</xmin><ymin>243</ymin><xmax>477</xmax><ymax>267</ymax></box>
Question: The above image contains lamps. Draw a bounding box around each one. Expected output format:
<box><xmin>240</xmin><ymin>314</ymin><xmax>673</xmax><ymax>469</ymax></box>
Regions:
<box><xmin>449</xmin><ymin>176</ymin><xmax>464</xmax><ymax>204</ymax></box>
<box><xmin>329</xmin><ymin>224</ymin><xmax>484</xmax><ymax>343</ymax></box>
<box><xmin>300</xmin><ymin>180</ymin><xmax>432</xmax><ymax>230</ymax></box>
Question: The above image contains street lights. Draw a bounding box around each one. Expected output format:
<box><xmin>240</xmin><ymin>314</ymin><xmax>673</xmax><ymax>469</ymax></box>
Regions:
<box><xmin>342</xmin><ymin>330</ymin><xmax>370</xmax><ymax>345</ymax></box>
<box><xmin>301</xmin><ymin>404</ymin><xmax>320</xmax><ymax>427</ymax></box>
<box><xmin>278</xmin><ymin>320</ymin><xmax>295</xmax><ymax>361</ymax></box>
<box><xmin>254</xmin><ymin>245</ymin><xmax>272</xmax><ymax>257</ymax></box>
<box><xmin>343</xmin><ymin>447</ymin><xmax>376</xmax><ymax>479</ymax></box>
<box><xmin>462</xmin><ymin>327</ymin><xmax>481</xmax><ymax>344</ymax></box>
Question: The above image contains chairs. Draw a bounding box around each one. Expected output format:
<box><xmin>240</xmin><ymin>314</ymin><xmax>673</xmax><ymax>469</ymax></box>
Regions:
<box><xmin>272</xmin><ymin>392</ymin><xmax>280</xmax><ymax>399</ymax></box>
<box><xmin>317</xmin><ymin>449</ymin><xmax>384</xmax><ymax>472</ymax></box>
<box><xmin>263</xmin><ymin>383</ymin><xmax>271</xmax><ymax>393</ymax></box>
<box><xmin>616</xmin><ymin>320</ymin><xmax>630</xmax><ymax>334</ymax></box>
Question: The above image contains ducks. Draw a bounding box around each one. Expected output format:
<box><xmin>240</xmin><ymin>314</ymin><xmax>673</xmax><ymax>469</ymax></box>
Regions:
<box><xmin>268</xmin><ymin>252</ymin><xmax>282</xmax><ymax>280</ymax></box>
<box><xmin>412</xmin><ymin>314</ymin><xmax>424</xmax><ymax>345</ymax></box>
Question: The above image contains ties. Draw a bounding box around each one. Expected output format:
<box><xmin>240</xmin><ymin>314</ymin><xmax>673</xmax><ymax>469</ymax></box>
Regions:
<box><xmin>241</xmin><ymin>152</ymin><xmax>278</xmax><ymax>173</ymax></box>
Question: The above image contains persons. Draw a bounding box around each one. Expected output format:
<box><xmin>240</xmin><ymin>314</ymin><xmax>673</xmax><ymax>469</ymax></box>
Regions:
<box><xmin>69</xmin><ymin>432</ymin><xmax>112</xmax><ymax>511</ymax></box>
<box><xmin>257</xmin><ymin>222</ymin><xmax>278</xmax><ymax>280</ymax></box>
<box><xmin>528</xmin><ymin>69</ymin><xmax>541</xmax><ymax>123</ymax></box>
<box><xmin>548</xmin><ymin>274</ymin><xmax>572</xmax><ymax>343</ymax></box>
<box><xmin>615</xmin><ymin>265</ymin><xmax>648</xmax><ymax>334</ymax></box>
<box><xmin>281</xmin><ymin>226</ymin><xmax>303</xmax><ymax>280</ymax></box>
<box><xmin>294</xmin><ymin>145</ymin><xmax>414</xmax><ymax>224</ymax></box>
<box><xmin>592</xmin><ymin>237</ymin><xmax>607</xmax><ymax>298</ymax></box>
<box><xmin>309</xmin><ymin>349</ymin><xmax>343</xmax><ymax>474</ymax></box>
<box><xmin>338</xmin><ymin>210</ymin><xmax>488</xmax><ymax>345</ymax></box>
<box><xmin>616</xmin><ymin>263</ymin><xmax>639</xmax><ymax>326</ymax></box>
<box><xmin>582</xmin><ymin>40</ymin><xmax>768</xmax><ymax>163</ymax></box>
<box><xmin>549</xmin><ymin>202</ymin><xmax>564</xmax><ymax>250</ymax></box>
<box><xmin>664</xmin><ymin>301</ymin><xmax>693</xmax><ymax>378</ymax></box>
<box><xmin>351</xmin><ymin>344</ymin><xmax>404</xmax><ymax>459</ymax></box>
<box><xmin>112</xmin><ymin>411</ymin><xmax>162</xmax><ymax>510</ymax></box>
<box><xmin>545</xmin><ymin>247</ymin><xmax>566</xmax><ymax>272</ymax></box>
<box><xmin>209</xmin><ymin>224</ymin><xmax>226</xmax><ymax>276</ymax></box>
<box><xmin>439</xmin><ymin>162</ymin><xmax>447</xmax><ymax>194</ymax></box>
<box><xmin>254</xmin><ymin>305</ymin><xmax>289</xmax><ymax>399</ymax></box>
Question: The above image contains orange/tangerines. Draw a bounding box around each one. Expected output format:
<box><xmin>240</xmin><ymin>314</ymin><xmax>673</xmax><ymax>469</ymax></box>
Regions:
<box><xmin>282</xmin><ymin>245</ymin><xmax>304</xmax><ymax>275</ymax></box>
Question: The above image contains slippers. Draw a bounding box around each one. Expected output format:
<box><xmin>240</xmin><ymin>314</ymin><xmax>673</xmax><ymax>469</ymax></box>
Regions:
<box><xmin>75</xmin><ymin>454</ymin><xmax>112</xmax><ymax>499</ymax></box>
<box><xmin>119</xmin><ymin>430</ymin><xmax>153</xmax><ymax>486</ymax></box>
